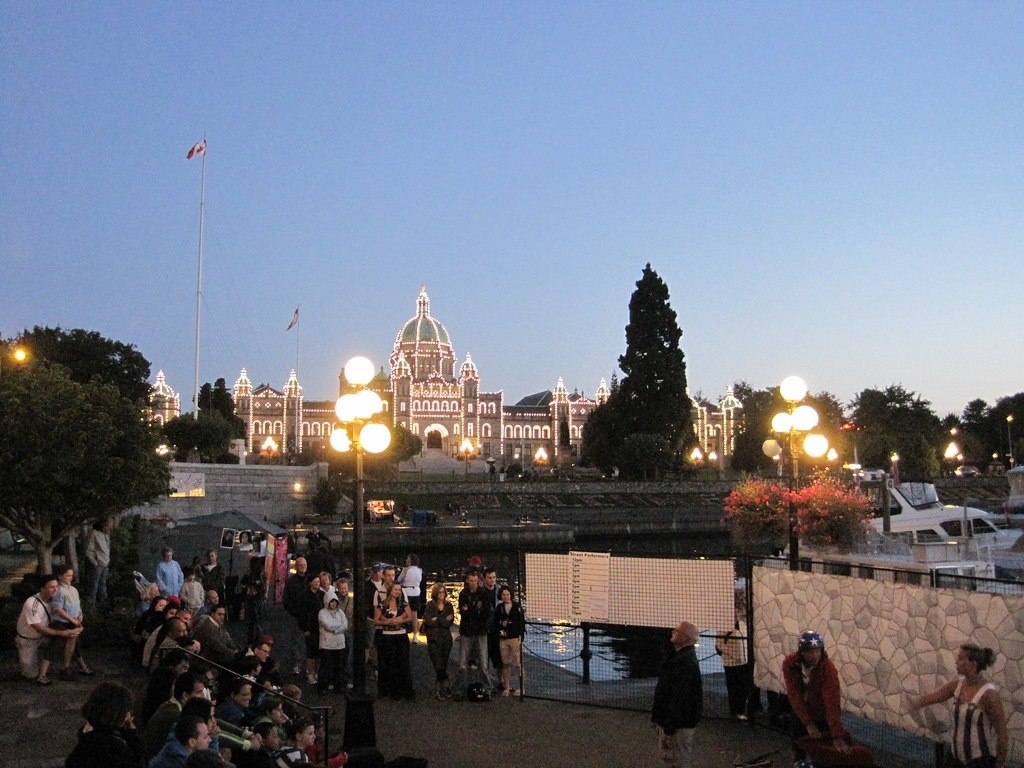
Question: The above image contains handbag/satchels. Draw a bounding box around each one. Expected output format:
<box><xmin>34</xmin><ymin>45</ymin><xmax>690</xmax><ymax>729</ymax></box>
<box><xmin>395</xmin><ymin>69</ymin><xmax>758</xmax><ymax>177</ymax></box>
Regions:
<box><xmin>419</xmin><ymin>622</ymin><xmax>434</xmax><ymax>636</ymax></box>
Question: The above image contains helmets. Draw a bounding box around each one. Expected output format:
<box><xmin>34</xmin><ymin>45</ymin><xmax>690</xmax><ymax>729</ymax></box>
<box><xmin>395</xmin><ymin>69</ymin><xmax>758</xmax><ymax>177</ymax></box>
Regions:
<box><xmin>797</xmin><ymin>630</ymin><xmax>825</xmax><ymax>661</ymax></box>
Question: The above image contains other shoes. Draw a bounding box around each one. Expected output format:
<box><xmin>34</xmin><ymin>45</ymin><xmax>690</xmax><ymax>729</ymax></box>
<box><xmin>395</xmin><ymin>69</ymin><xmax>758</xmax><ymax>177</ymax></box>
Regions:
<box><xmin>437</xmin><ymin>681</ymin><xmax>453</xmax><ymax>701</ymax></box>
<box><xmin>503</xmin><ymin>687</ymin><xmax>525</xmax><ymax>696</ymax></box>
<box><xmin>306</xmin><ymin>668</ymin><xmax>318</xmax><ymax>683</ymax></box>
<box><xmin>79</xmin><ymin>667</ymin><xmax>96</xmax><ymax>676</ymax></box>
<box><xmin>35</xmin><ymin>674</ymin><xmax>52</xmax><ymax>685</ymax></box>
<box><xmin>59</xmin><ymin>666</ymin><xmax>73</xmax><ymax>679</ymax></box>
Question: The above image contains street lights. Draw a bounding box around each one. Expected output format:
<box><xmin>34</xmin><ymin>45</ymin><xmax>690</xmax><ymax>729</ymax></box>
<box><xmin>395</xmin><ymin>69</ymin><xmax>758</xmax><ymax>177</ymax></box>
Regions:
<box><xmin>329</xmin><ymin>352</ymin><xmax>389</xmax><ymax>768</ymax></box>
<box><xmin>763</xmin><ymin>374</ymin><xmax>828</xmax><ymax>572</ymax></box>
<box><xmin>1007</xmin><ymin>414</ymin><xmax>1013</xmax><ymax>453</ymax></box>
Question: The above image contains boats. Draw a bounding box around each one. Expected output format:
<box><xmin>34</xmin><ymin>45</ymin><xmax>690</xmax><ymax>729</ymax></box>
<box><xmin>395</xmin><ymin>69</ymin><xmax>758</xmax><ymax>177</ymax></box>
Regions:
<box><xmin>782</xmin><ymin>464</ymin><xmax>1024</xmax><ymax>597</ymax></box>
<box><xmin>436</xmin><ymin>558</ymin><xmax>506</xmax><ymax>583</ymax></box>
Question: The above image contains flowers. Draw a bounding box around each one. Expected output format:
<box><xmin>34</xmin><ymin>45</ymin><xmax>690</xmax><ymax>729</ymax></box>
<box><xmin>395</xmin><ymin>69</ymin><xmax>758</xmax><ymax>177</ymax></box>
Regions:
<box><xmin>719</xmin><ymin>468</ymin><xmax>875</xmax><ymax>550</ymax></box>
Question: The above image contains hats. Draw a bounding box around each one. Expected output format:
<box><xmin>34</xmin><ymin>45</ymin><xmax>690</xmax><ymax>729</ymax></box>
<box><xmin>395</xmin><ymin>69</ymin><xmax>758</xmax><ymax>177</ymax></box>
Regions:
<box><xmin>467</xmin><ymin>556</ymin><xmax>481</xmax><ymax>565</ymax></box>
<box><xmin>371</xmin><ymin>562</ymin><xmax>382</xmax><ymax>572</ymax></box>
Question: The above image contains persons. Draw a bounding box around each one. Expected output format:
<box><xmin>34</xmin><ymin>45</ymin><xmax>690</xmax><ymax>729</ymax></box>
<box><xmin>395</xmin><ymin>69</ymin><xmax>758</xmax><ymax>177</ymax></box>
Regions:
<box><xmin>82</xmin><ymin>518</ymin><xmax>115</xmax><ymax>605</ymax></box>
<box><xmin>304</xmin><ymin>522</ymin><xmax>330</xmax><ymax>551</ymax></box>
<box><xmin>238</xmin><ymin>531</ymin><xmax>253</xmax><ymax>550</ymax></box>
<box><xmin>249</xmin><ymin>532</ymin><xmax>266</xmax><ymax>584</ymax></box>
<box><xmin>649</xmin><ymin>621</ymin><xmax>705</xmax><ymax>768</ymax></box>
<box><xmin>13</xmin><ymin>565</ymin><xmax>97</xmax><ymax>687</ymax></box>
<box><xmin>487</xmin><ymin>462</ymin><xmax>538</xmax><ymax>484</ymax></box>
<box><xmin>63</xmin><ymin>547</ymin><xmax>527</xmax><ymax>768</ymax></box>
<box><xmin>715</xmin><ymin>604</ymin><xmax>751</xmax><ymax>721</ymax></box>
<box><xmin>904</xmin><ymin>641</ymin><xmax>1011</xmax><ymax>768</ymax></box>
<box><xmin>781</xmin><ymin>630</ymin><xmax>854</xmax><ymax>768</ymax></box>
<box><xmin>222</xmin><ymin>529</ymin><xmax>234</xmax><ymax>548</ymax></box>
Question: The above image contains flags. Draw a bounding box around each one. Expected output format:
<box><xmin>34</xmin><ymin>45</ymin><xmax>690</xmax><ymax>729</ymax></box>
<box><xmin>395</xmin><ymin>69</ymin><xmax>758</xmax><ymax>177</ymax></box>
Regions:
<box><xmin>186</xmin><ymin>140</ymin><xmax>207</xmax><ymax>160</ymax></box>
<box><xmin>284</xmin><ymin>308</ymin><xmax>300</xmax><ymax>331</ymax></box>
